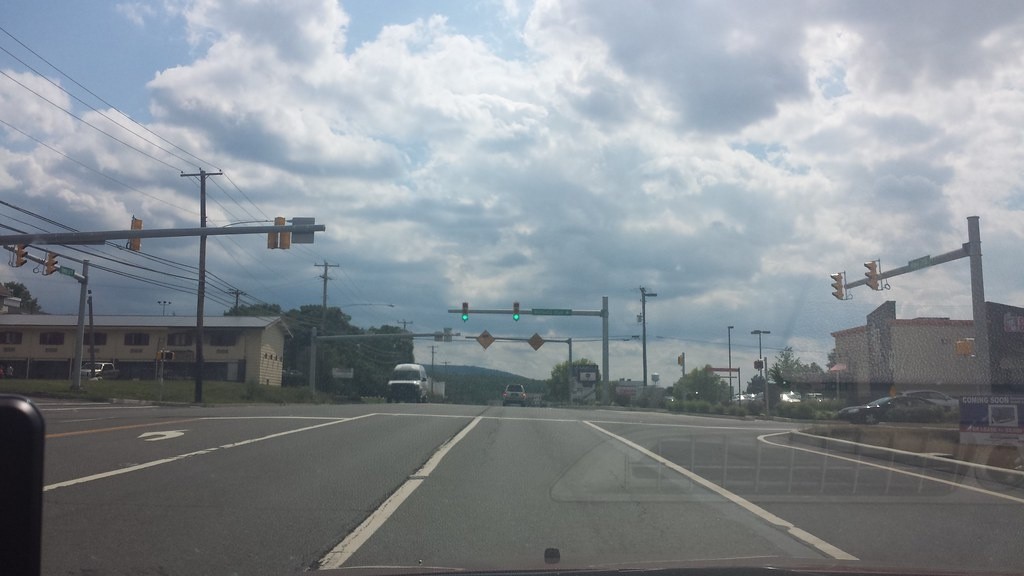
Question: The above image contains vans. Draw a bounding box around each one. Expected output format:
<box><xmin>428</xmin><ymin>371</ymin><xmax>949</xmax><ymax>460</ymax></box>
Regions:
<box><xmin>388</xmin><ymin>364</ymin><xmax>429</xmax><ymax>401</ymax></box>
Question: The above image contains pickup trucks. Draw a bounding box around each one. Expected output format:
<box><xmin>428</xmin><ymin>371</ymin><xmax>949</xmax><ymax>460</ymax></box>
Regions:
<box><xmin>504</xmin><ymin>384</ymin><xmax>527</xmax><ymax>408</ymax></box>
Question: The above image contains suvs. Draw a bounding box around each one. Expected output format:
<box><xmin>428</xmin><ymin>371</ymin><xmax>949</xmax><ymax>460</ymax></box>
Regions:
<box><xmin>897</xmin><ymin>389</ymin><xmax>959</xmax><ymax>414</ymax></box>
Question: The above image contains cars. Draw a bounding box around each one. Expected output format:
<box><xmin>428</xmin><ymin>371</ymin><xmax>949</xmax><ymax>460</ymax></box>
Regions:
<box><xmin>836</xmin><ymin>395</ymin><xmax>945</xmax><ymax>426</ymax></box>
<box><xmin>728</xmin><ymin>390</ymin><xmax>824</xmax><ymax>404</ymax></box>
<box><xmin>71</xmin><ymin>362</ymin><xmax>120</xmax><ymax>382</ymax></box>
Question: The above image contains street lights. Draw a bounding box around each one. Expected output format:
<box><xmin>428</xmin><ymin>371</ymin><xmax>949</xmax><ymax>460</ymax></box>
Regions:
<box><xmin>751</xmin><ymin>329</ymin><xmax>771</xmax><ymax>376</ymax></box>
<box><xmin>642</xmin><ymin>292</ymin><xmax>657</xmax><ymax>389</ymax></box>
<box><xmin>318</xmin><ymin>303</ymin><xmax>395</xmax><ymax>392</ymax></box>
<box><xmin>726</xmin><ymin>325</ymin><xmax>734</xmax><ymax>408</ymax></box>
<box><xmin>157</xmin><ymin>298</ymin><xmax>174</xmax><ymax>317</ymax></box>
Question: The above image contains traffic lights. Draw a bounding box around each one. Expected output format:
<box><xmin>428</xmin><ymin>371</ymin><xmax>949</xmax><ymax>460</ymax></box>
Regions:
<box><xmin>678</xmin><ymin>356</ymin><xmax>683</xmax><ymax>364</ymax></box>
<box><xmin>167</xmin><ymin>352</ymin><xmax>174</xmax><ymax>360</ymax></box>
<box><xmin>15</xmin><ymin>245</ymin><xmax>29</xmax><ymax>267</ymax></box>
<box><xmin>514</xmin><ymin>302</ymin><xmax>520</xmax><ymax>320</ymax></box>
<box><xmin>158</xmin><ymin>350</ymin><xmax>166</xmax><ymax>360</ymax></box>
<box><xmin>864</xmin><ymin>260</ymin><xmax>879</xmax><ymax>291</ymax></box>
<box><xmin>45</xmin><ymin>253</ymin><xmax>60</xmax><ymax>275</ymax></box>
<box><xmin>462</xmin><ymin>302</ymin><xmax>469</xmax><ymax>322</ymax></box>
<box><xmin>828</xmin><ymin>273</ymin><xmax>845</xmax><ymax>302</ymax></box>
